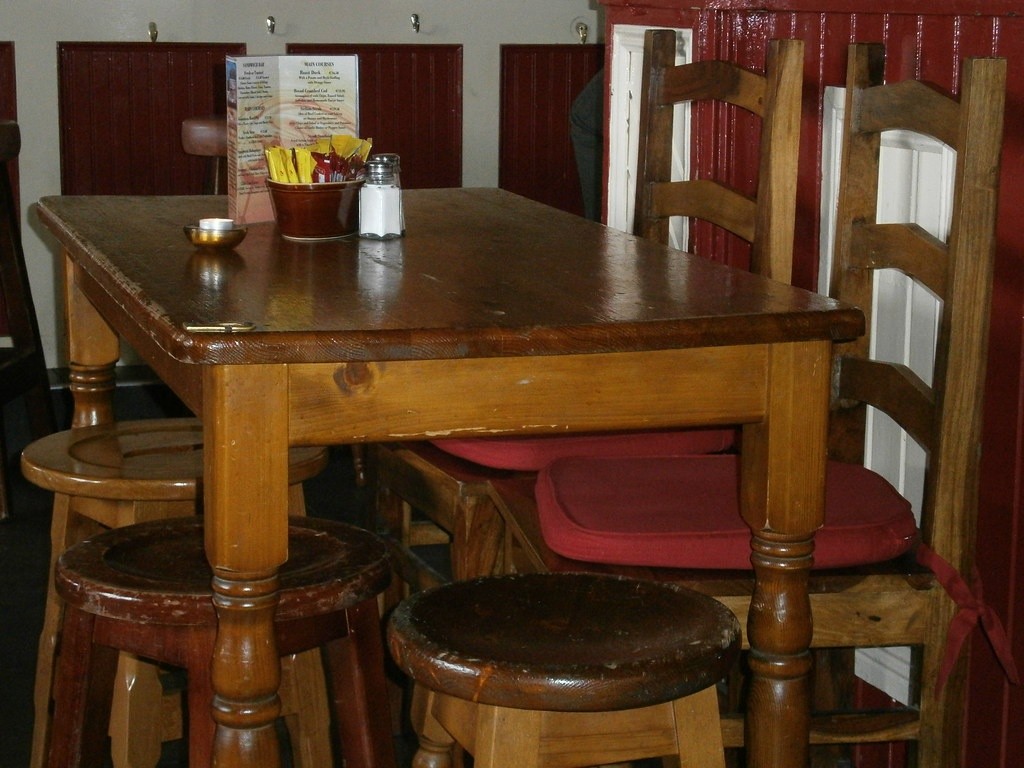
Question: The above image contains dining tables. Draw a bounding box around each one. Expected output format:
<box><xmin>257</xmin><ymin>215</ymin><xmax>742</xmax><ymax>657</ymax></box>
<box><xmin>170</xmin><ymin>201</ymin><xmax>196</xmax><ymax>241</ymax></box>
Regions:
<box><xmin>29</xmin><ymin>185</ymin><xmax>867</xmax><ymax>767</ymax></box>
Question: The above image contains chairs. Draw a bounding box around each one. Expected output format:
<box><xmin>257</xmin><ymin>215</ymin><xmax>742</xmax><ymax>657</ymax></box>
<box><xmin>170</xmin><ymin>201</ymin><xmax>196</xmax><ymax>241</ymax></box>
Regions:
<box><xmin>0</xmin><ymin>120</ymin><xmax>189</xmax><ymax>516</ymax></box>
<box><xmin>371</xmin><ymin>30</ymin><xmax>807</xmax><ymax>609</ymax></box>
<box><xmin>467</xmin><ymin>38</ymin><xmax>1011</xmax><ymax>767</ymax></box>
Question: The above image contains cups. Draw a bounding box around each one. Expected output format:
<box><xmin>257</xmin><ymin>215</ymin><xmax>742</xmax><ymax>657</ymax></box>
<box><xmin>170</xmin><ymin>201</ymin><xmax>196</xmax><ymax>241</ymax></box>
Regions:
<box><xmin>199</xmin><ymin>218</ymin><xmax>233</xmax><ymax>231</ymax></box>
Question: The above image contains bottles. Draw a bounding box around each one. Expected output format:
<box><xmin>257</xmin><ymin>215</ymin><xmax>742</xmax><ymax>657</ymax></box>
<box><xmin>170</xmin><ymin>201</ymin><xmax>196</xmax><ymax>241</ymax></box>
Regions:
<box><xmin>368</xmin><ymin>154</ymin><xmax>406</xmax><ymax>235</ymax></box>
<box><xmin>357</xmin><ymin>159</ymin><xmax>403</xmax><ymax>242</ymax></box>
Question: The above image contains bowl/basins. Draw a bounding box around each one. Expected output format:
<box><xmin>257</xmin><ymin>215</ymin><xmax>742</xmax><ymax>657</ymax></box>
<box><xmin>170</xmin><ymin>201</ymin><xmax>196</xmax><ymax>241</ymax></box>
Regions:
<box><xmin>264</xmin><ymin>174</ymin><xmax>366</xmax><ymax>239</ymax></box>
<box><xmin>182</xmin><ymin>225</ymin><xmax>248</xmax><ymax>253</ymax></box>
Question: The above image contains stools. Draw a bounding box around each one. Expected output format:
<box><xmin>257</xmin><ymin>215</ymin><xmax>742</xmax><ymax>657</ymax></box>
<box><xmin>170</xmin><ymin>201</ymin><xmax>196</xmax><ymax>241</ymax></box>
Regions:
<box><xmin>34</xmin><ymin>511</ymin><xmax>406</xmax><ymax>768</ymax></box>
<box><xmin>385</xmin><ymin>565</ymin><xmax>744</xmax><ymax>768</ymax></box>
<box><xmin>13</xmin><ymin>412</ymin><xmax>335</xmax><ymax>765</ymax></box>
